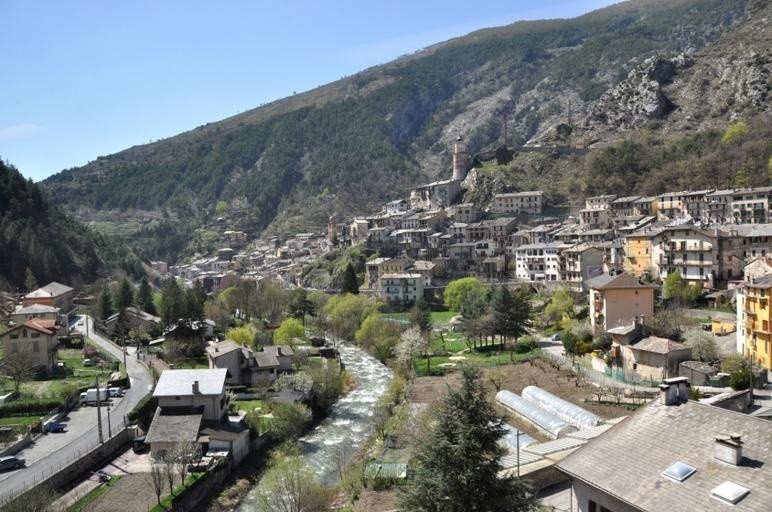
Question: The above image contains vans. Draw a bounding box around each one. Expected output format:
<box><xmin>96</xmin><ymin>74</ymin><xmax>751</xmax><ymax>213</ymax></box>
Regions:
<box><xmin>108</xmin><ymin>388</ymin><xmax>121</xmax><ymax>397</ymax></box>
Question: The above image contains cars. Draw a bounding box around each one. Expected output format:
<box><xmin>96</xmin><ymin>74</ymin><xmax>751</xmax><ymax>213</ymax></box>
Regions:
<box><xmin>0</xmin><ymin>456</ymin><xmax>25</xmax><ymax>474</ymax></box>
<box><xmin>57</xmin><ymin>362</ymin><xmax>64</xmax><ymax>372</ymax></box>
<box><xmin>78</xmin><ymin>321</ymin><xmax>83</xmax><ymax>325</ymax></box>
<box><xmin>84</xmin><ymin>358</ymin><xmax>91</xmax><ymax>367</ymax></box>
<box><xmin>551</xmin><ymin>333</ymin><xmax>562</xmax><ymax>341</ymax></box>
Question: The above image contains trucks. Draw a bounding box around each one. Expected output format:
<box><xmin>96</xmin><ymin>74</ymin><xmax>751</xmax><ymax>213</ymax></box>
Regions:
<box><xmin>79</xmin><ymin>388</ymin><xmax>110</xmax><ymax>407</ymax></box>
<box><xmin>48</xmin><ymin>419</ymin><xmax>68</xmax><ymax>433</ymax></box>
